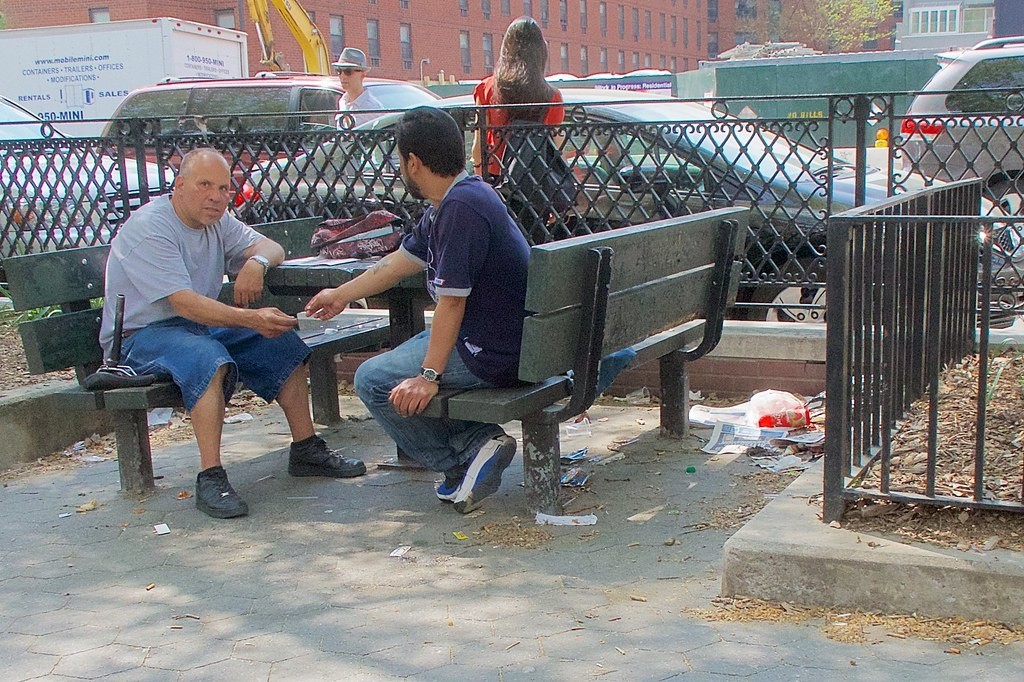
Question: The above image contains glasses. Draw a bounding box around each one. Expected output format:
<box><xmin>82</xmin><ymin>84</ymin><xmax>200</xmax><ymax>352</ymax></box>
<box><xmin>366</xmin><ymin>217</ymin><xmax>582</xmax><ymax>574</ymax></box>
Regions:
<box><xmin>335</xmin><ymin>68</ymin><xmax>363</xmax><ymax>76</ymax></box>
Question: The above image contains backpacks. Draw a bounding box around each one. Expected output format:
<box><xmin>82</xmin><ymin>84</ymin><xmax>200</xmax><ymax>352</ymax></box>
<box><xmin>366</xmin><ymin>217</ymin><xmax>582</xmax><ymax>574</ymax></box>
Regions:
<box><xmin>501</xmin><ymin>87</ymin><xmax>579</xmax><ymax>214</ymax></box>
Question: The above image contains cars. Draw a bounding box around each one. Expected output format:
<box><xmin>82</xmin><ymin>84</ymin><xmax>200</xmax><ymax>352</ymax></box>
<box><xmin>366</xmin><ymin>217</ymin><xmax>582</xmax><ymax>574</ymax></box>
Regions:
<box><xmin>1</xmin><ymin>97</ymin><xmax>184</xmax><ymax>270</ymax></box>
<box><xmin>235</xmin><ymin>86</ymin><xmax>1024</xmax><ymax>331</ymax></box>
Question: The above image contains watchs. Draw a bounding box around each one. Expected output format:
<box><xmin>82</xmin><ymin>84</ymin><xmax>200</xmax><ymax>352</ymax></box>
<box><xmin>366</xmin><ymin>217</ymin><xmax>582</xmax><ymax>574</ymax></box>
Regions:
<box><xmin>248</xmin><ymin>255</ymin><xmax>268</xmax><ymax>277</ymax></box>
<box><xmin>419</xmin><ymin>367</ymin><xmax>442</xmax><ymax>382</ymax></box>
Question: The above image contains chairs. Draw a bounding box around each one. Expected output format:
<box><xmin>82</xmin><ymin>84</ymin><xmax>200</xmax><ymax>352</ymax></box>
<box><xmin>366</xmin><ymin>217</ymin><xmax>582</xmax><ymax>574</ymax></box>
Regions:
<box><xmin>565</xmin><ymin>136</ymin><xmax>612</xmax><ymax>185</ymax></box>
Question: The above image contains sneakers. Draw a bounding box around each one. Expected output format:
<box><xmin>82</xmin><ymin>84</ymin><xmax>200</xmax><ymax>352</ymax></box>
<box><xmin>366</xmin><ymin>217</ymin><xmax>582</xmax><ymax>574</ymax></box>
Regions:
<box><xmin>196</xmin><ymin>469</ymin><xmax>249</xmax><ymax>519</ymax></box>
<box><xmin>288</xmin><ymin>436</ymin><xmax>366</xmax><ymax>478</ymax></box>
<box><xmin>436</xmin><ymin>432</ymin><xmax>517</xmax><ymax>515</ymax></box>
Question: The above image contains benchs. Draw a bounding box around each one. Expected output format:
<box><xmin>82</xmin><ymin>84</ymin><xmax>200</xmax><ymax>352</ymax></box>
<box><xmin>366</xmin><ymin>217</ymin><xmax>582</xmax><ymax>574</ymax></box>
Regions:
<box><xmin>0</xmin><ymin>216</ymin><xmax>389</xmax><ymax>494</ymax></box>
<box><xmin>412</xmin><ymin>207</ymin><xmax>751</xmax><ymax>514</ymax></box>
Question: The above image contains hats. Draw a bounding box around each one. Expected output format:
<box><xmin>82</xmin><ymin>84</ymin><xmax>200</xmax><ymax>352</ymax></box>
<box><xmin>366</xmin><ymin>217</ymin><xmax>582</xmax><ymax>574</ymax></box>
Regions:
<box><xmin>331</xmin><ymin>47</ymin><xmax>371</xmax><ymax>74</ymax></box>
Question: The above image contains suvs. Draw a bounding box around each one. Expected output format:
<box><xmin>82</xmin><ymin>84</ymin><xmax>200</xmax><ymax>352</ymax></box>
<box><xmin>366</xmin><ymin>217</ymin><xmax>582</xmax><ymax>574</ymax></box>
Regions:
<box><xmin>899</xmin><ymin>36</ymin><xmax>1024</xmax><ymax>263</ymax></box>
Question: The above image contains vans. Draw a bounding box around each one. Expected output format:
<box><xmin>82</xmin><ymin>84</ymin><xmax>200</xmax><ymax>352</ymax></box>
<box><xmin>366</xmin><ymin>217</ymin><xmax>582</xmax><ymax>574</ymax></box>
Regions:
<box><xmin>101</xmin><ymin>71</ymin><xmax>444</xmax><ymax>209</ymax></box>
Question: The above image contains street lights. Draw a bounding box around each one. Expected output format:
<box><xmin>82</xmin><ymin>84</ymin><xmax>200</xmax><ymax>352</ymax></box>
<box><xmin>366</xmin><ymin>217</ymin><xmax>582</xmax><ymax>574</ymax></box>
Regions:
<box><xmin>420</xmin><ymin>58</ymin><xmax>430</xmax><ymax>82</ymax></box>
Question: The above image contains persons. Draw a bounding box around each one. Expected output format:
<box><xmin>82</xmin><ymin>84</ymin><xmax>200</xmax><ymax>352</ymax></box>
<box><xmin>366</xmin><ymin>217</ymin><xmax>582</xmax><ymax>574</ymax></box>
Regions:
<box><xmin>472</xmin><ymin>17</ymin><xmax>565</xmax><ymax>246</ymax></box>
<box><xmin>331</xmin><ymin>47</ymin><xmax>385</xmax><ymax>130</ymax></box>
<box><xmin>98</xmin><ymin>145</ymin><xmax>366</xmax><ymax>518</ymax></box>
<box><xmin>303</xmin><ymin>105</ymin><xmax>531</xmax><ymax>513</ymax></box>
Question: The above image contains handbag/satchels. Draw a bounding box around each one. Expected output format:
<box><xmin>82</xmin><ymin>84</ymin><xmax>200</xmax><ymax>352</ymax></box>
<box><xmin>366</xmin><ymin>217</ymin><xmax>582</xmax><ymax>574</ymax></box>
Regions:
<box><xmin>309</xmin><ymin>209</ymin><xmax>405</xmax><ymax>261</ymax></box>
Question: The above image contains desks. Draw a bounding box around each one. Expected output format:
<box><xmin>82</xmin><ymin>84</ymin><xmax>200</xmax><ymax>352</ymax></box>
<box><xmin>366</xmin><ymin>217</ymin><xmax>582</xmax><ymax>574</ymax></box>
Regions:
<box><xmin>266</xmin><ymin>256</ymin><xmax>430</xmax><ymax>472</ymax></box>
